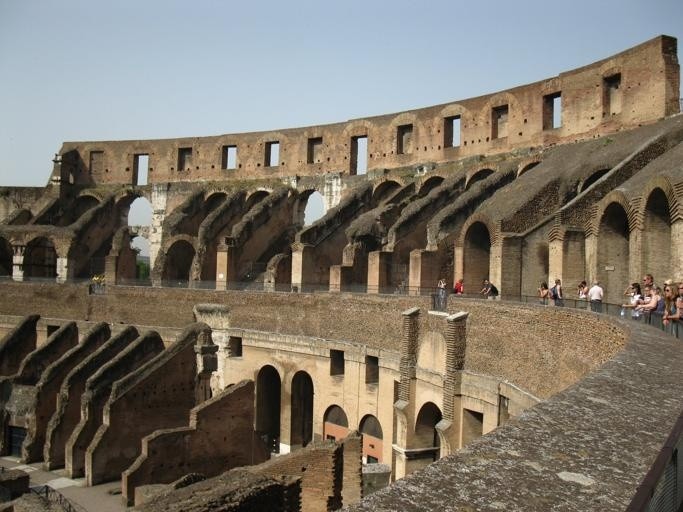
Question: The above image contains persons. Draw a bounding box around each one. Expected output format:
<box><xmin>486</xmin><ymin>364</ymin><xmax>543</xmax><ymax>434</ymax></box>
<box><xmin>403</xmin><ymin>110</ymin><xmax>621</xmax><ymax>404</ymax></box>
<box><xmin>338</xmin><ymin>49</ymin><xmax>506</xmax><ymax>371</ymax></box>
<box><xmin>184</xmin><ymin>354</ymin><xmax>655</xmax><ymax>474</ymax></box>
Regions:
<box><xmin>587</xmin><ymin>280</ymin><xmax>604</xmax><ymax>313</ymax></box>
<box><xmin>549</xmin><ymin>279</ymin><xmax>566</xmax><ymax>307</ymax></box>
<box><xmin>576</xmin><ymin>281</ymin><xmax>589</xmax><ymax>309</ymax></box>
<box><xmin>620</xmin><ymin>274</ymin><xmax>683</xmax><ymax>327</ymax></box>
<box><xmin>479</xmin><ymin>279</ymin><xmax>498</xmax><ymax>299</ymax></box>
<box><xmin>454</xmin><ymin>278</ymin><xmax>465</xmax><ymax>294</ymax></box>
<box><xmin>438</xmin><ymin>278</ymin><xmax>447</xmax><ymax>302</ymax></box>
<box><xmin>92</xmin><ymin>274</ymin><xmax>105</xmax><ymax>285</ymax></box>
<box><xmin>537</xmin><ymin>280</ymin><xmax>548</xmax><ymax>304</ymax></box>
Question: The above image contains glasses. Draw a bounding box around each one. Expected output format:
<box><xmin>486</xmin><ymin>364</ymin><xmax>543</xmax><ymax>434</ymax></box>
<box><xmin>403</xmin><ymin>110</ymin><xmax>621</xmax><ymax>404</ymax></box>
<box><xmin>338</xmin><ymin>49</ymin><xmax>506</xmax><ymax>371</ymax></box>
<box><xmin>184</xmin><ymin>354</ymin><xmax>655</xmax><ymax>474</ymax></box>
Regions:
<box><xmin>643</xmin><ymin>279</ymin><xmax>648</xmax><ymax>281</ymax></box>
<box><xmin>632</xmin><ymin>288</ymin><xmax>635</xmax><ymax>289</ymax></box>
<box><xmin>664</xmin><ymin>290</ymin><xmax>671</xmax><ymax>292</ymax></box>
<box><xmin>664</xmin><ymin>284</ymin><xmax>668</xmax><ymax>286</ymax></box>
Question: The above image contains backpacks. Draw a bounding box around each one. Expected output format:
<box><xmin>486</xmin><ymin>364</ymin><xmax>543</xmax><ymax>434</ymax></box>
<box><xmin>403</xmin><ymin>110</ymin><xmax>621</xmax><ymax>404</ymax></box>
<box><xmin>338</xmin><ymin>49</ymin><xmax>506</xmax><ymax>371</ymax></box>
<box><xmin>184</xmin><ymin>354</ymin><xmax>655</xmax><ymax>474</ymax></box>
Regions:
<box><xmin>549</xmin><ymin>286</ymin><xmax>557</xmax><ymax>300</ymax></box>
